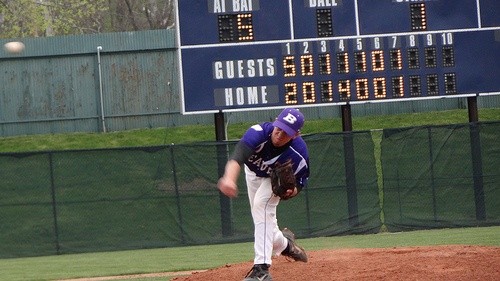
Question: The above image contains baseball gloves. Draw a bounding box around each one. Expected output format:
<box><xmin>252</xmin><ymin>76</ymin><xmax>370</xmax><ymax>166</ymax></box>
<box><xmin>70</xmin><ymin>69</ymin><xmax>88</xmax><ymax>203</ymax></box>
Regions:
<box><xmin>271</xmin><ymin>160</ymin><xmax>297</xmax><ymax>199</ymax></box>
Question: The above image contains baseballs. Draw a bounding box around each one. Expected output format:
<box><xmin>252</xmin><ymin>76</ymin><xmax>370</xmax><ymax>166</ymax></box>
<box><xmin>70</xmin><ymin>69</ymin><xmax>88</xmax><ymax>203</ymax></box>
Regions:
<box><xmin>6</xmin><ymin>42</ymin><xmax>24</xmax><ymax>53</ymax></box>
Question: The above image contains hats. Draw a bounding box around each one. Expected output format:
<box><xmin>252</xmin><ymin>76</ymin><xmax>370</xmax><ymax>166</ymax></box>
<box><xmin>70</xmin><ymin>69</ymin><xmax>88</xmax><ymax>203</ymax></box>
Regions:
<box><xmin>271</xmin><ymin>108</ymin><xmax>305</xmax><ymax>137</ymax></box>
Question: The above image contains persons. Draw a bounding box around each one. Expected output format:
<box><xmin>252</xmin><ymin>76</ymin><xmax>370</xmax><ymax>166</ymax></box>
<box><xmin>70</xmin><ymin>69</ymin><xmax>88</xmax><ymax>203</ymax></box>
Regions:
<box><xmin>217</xmin><ymin>108</ymin><xmax>310</xmax><ymax>281</ymax></box>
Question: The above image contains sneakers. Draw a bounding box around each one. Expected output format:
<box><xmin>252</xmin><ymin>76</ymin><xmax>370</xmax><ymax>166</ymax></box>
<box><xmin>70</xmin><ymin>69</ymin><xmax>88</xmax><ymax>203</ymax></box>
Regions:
<box><xmin>280</xmin><ymin>229</ymin><xmax>307</xmax><ymax>263</ymax></box>
<box><xmin>242</xmin><ymin>263</ymin><xmax>270</xmax><ymax>281</ymax></box>
